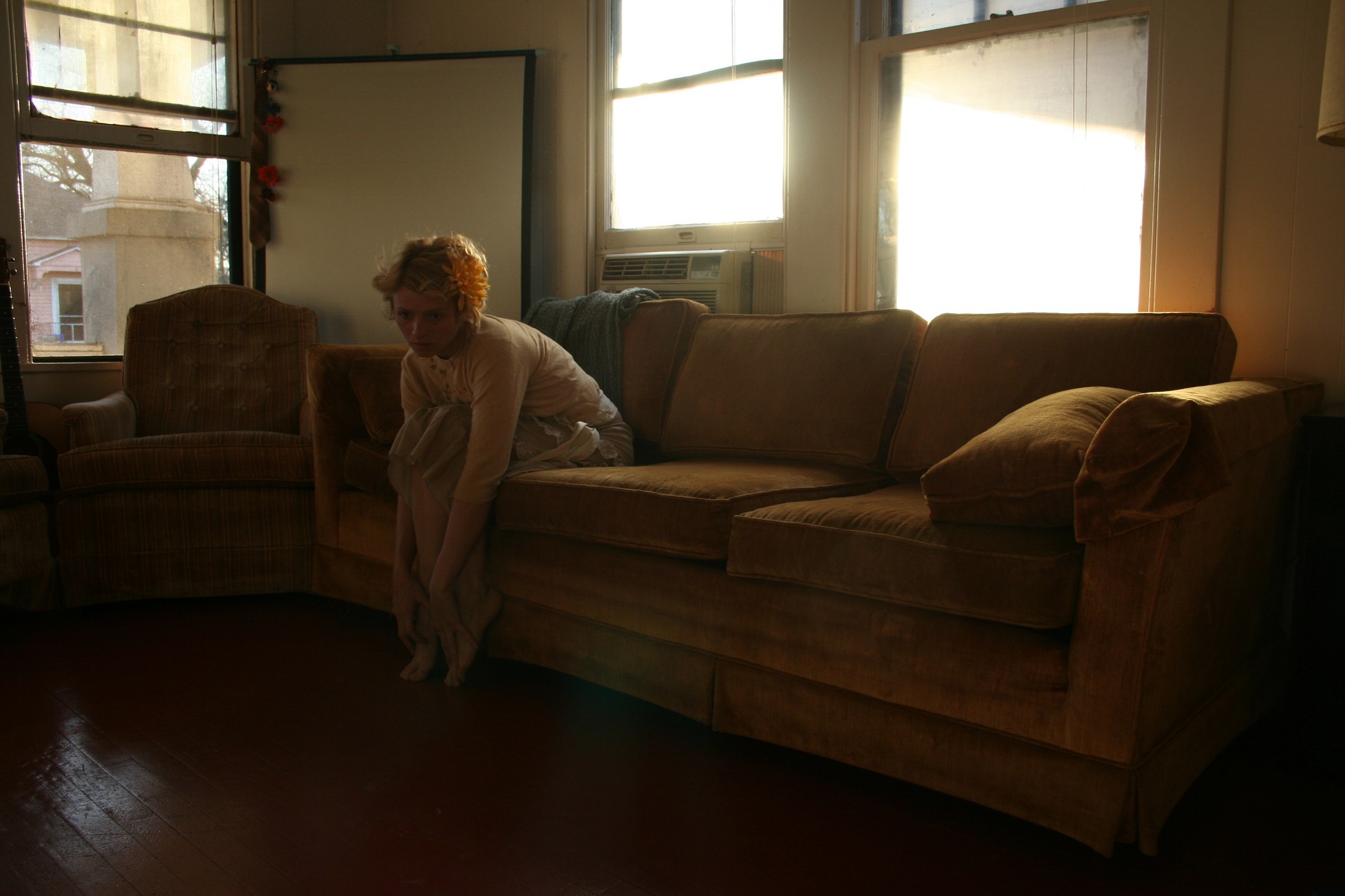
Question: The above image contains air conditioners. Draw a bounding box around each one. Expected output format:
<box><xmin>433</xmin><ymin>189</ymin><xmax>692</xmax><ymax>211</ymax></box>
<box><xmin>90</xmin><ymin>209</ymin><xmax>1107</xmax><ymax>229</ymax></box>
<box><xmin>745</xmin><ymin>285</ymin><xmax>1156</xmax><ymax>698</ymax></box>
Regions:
<box><xmin>598</xmin><ymin>249</ymin><xmax>739</xmax><ymax>313</ymax></box>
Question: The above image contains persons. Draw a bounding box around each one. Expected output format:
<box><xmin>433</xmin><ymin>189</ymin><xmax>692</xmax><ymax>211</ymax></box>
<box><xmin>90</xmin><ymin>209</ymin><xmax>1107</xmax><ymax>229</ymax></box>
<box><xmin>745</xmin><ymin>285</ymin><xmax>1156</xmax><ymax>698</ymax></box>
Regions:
<box><xmin>372</xmin><ymin>234</ymin><xmax>633</xmax><ymax>684</ymax></box>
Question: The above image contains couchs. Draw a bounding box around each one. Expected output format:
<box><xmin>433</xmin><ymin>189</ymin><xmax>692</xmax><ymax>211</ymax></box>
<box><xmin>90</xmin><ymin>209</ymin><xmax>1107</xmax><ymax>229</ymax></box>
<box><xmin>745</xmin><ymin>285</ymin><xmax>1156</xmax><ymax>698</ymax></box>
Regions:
<box><xmin>299</xmin><ymin>298</ymin><xmax>1331</xmax><ymax>859</ymax></box>
<box><xmin>58</xmin><ymin>280</ymin><xmax>319</xmax><ymax>610</ymax></box>
<box><xmin>0</xmin><ymin>411</ymin><xmax>59</xmax><ymax>610</ymax></box>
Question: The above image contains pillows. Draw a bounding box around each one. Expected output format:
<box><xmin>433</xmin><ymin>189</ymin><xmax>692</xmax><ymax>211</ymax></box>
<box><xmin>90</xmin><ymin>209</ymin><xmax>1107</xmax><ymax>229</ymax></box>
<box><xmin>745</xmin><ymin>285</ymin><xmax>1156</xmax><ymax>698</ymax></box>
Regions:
<box><xmin>344</xmin><ymin>355</ymin><xmax>405</xmax><ymax>439</ymax></box>
<box><xmin>921</xmin><ymin>385</ymin><xmax>1140</xmax><ymax>533</ymax></box>
